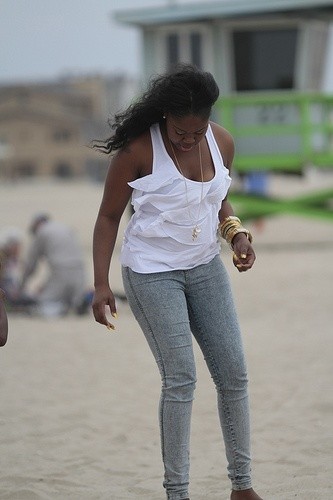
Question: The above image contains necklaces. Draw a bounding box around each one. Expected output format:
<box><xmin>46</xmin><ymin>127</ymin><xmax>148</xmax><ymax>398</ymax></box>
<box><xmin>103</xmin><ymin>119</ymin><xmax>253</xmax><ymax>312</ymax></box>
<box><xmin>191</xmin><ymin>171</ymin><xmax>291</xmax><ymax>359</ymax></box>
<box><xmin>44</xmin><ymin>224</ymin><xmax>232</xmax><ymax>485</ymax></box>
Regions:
<box><xmin>170</xmin><ymin>142</ymin><xmax>204</xmax><ymax>242</ymax></box>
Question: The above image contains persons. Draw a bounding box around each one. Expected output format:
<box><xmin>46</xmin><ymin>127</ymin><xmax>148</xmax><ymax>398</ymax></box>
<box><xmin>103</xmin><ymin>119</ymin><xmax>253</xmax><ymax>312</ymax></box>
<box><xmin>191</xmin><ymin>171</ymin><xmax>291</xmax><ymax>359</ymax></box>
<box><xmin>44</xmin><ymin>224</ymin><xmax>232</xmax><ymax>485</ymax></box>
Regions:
<box><xmin>0</xmin><ymin>214</ymin><xmax>87</xmax><ymax>347</ymax></box>
<box><xmin>92</xmin><ymin>64</ymin><xmax>263</xmax><ymax>500</ymax></box>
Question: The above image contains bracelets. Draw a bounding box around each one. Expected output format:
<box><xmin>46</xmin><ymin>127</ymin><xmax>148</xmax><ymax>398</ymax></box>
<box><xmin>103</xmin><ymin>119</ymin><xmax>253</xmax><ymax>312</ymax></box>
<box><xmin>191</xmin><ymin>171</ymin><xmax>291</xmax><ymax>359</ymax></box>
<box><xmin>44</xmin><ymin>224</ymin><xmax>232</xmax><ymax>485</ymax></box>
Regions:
<box><xmin>218</xmin><ymin>216</ymin><xmax>252</xmax><ymax>250</ymax></box>
